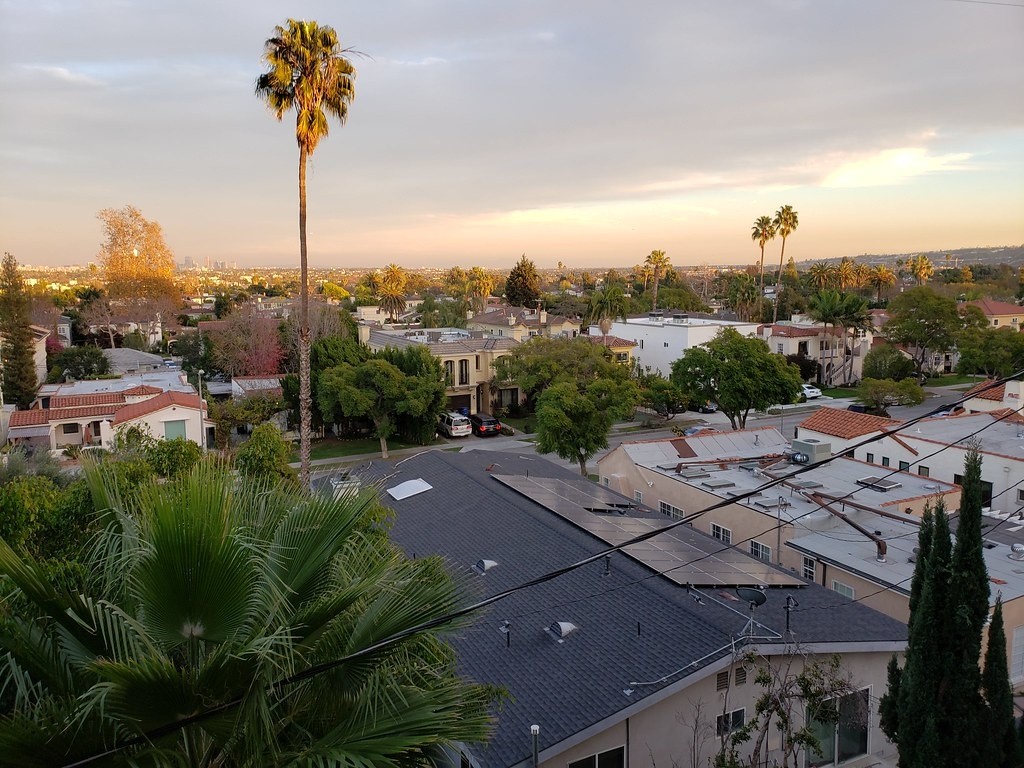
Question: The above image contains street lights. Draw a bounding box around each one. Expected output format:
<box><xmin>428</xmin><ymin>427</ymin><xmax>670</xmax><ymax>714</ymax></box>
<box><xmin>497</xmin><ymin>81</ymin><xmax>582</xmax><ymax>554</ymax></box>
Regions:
<box><xmin>199</xmin><ymin>369</ymin><xmax>205</xmax><ymax>451</ymax></box>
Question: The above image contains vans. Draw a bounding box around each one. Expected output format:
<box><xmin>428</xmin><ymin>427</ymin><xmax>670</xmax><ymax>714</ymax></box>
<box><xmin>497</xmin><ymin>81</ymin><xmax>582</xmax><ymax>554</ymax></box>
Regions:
<box><xmin>439</xmin><ymin>413</ymin><xmax>472</xmax><ymax>439</ymax></box>
<box><xmin>848</xmin><ymin>403</ymin><xmax>891</xmax><ymax>418</ymax></box>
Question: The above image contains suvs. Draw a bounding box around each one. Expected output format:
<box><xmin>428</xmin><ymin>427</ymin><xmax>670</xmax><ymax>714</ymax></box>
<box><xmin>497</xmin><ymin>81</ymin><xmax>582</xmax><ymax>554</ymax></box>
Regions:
<box><xmin>469</xmin><ymin>413</ymin><xmax>502</xmax><ymax>437</ymax></box>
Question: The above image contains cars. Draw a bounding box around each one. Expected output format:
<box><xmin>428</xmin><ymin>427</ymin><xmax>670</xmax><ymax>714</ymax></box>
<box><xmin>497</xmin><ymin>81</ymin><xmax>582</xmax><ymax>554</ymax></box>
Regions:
<box><xmin>882</xmin><ymin>392</ymin><xmax>912</xmax><ymax>406</ymax></box>
<box><xmin>930</xmin><ymin>404</ymin><xmax>964</xmax><ymax>419</ymax></box>
<box><xmin>910</xmin><ymin>372</ymin><xmax>928</xmax><ymax>387</ymax></box>
<box><xmin>795</xmin><ymin>384</ymin><xmax>823</xmax><ymax>399</ymax></box>
<box><xmin>688</xmin><ymin>400</ymin><xmax>719</xmax><ymax>413</ymax></box>
<box><xmin>684</xmin><ymin>426</ymin><xmax>720</xmax><ymax>436</ymax></box>
